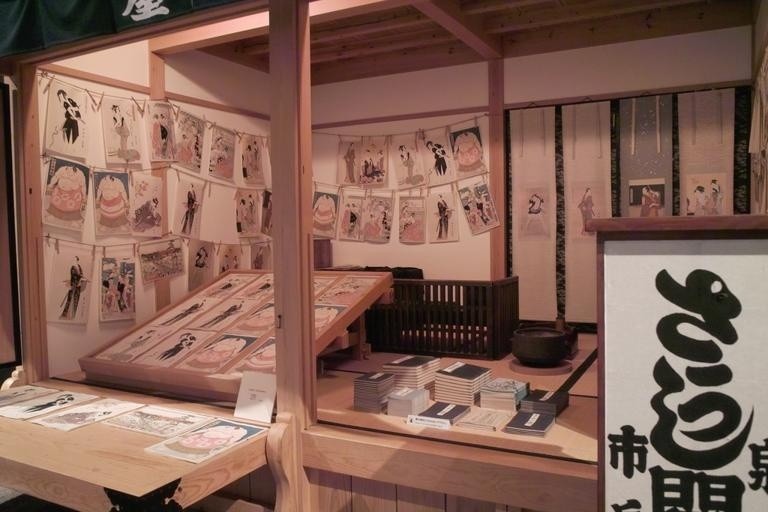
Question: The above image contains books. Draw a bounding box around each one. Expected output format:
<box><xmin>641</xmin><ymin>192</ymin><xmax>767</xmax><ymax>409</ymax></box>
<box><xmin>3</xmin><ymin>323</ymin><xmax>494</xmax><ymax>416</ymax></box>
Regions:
<box><xmin>503</xmin><ymin>411</ymin><xmax>555</xmax><ymax>436</ymax></box>
<box><xmin>435</xmin><ymin>361</ymin><xmax>494</xmax><ymax>406</ymax></box>
<box><xmin>519</xmin><ymin>389</ymin><xmax>569</xmax><ymax>416</ymax></box>
<box><xmin>410</xmin><ymin>401</ymin><xmax>471</xmax><ymax>430</ymax></box>
<box><xmin>387</xmin><ymin>386</ymin><xmax>430</xmax><ymax>417</ymax></box>
<box><xmin>383</xmin><ymin>354</ymin><xmax>440</xmax><ymax>390</ymax></box>
<box><xmin>353</xmin><ymin>372</ymin><xmax>395</xmax><ymax>414</ymax></box>
<box><xmin>457</xmin><ymin>408</ymin><xmax>512</xmax><ymax>433</ymax></box>
<box><xmin>480</xmin><ymin>377</ymin><xmax>530</xmax><ymax>411</ymax></box>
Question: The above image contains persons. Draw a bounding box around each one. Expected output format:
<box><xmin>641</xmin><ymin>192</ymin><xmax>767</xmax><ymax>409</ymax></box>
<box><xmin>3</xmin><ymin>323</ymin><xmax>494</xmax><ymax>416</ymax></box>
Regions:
<box><xmin>47</xmin><ymin>165</ymin><xmax>86</xmax><ymax>221</ymax></box>
<box><xmin>345</xmin><ymin>142</ymin><xmax>356</xmax><ymax>183</ymax></box>
<box><xmin>118</xmin><ymin>270</ymin><xmax>134</xmax><ymax>313</ymax></box>
<box><xmin>163</xmin><ymin>425</ymin><xmax>248</xmax><ymax>455</ymax></box>
<box><xmin>400</xmin><ymin>201</ymin><xmax>416</xmax><ymax>241</ymax></box>
<box><xmin>247</xmin><ymin>194</ymin><xmax>258</xmax><ymax>233</ymax></box>
<box><xmin>95</xmin><ymin>175</ymin><xmax>129</xmax><ymax>224</ymax></box>
<box><xmin>44</xmin><ymin>411</ymin><xmax>111</xmax><ymax>425</ymax></box>
<box><xmin>711</xmin><ymin>176</ymin><xmax>723</xmax><ymax>215</ymax></box>
<box><xmin>152</xmin><ymin>112</ymin><xmax>161</xmax><ymax>157</ymax></box>
<box><xmin>57</xmin><ymin>89</ymin><xmax>82</xmax><ymax>144</ymax></box>
<box><xmin>579</xmin><ymin>187</ymin><xmax>595</xmax><ymax>235</ymax></box>
<box><xmin>182</xmin><ymin>184</ymin><xmax>200</xmax><ymax>236</ymax></box>
<box><xmin>112</xmin><ymin>104</ymin><xmax>130</xmax><ymax>151</ymax></box>
<box><xmin>396</xmin><ymin>145</ymin><xmax>423</xmax><ymax>184</ymax></box>
<box><xmin>221</xmin><ymin>248</ymin><xmax>238</xmax><ymax>273</ymax></box>
<box><xmin>523</xmin><ymin>192</ymin><xmax>549</xmax><ymax>231</ymax></box>
<box><xmin>242</xmin><ymin>143</ymin><xmax>253</xmax><ymax>177</ymax></box>
<box><xmin>182</xmin><ymin>135</ymin><xmax>192</xmax><ymax>159</ymax></box>
<box><xmin>361</xmin><ymin>148</ymin><xmax>374</xmax><ymax>181</ymax></box>
<box><xmin>25</xmin><ymin>394</ymin><xmax>73</xmax><ymax>413</ymax></box>
<box><xmin>687</xmin><ymin>186</ymin><xmax>707</xmax><ymax>214</ymax></box>
<box><xmin>253</xmin><ymin>246</ymin><xmax>265</xmax><ymax>269</ymax></box>
<box><xmin>238</xmin><ymin>198</ymin><xmax>248</xmax><ymax>232</ymax></box>
<box><xmin>166</xmin><ymin>242</ymin><xmax>179</xmax><ymax>273</ymax></box>
<box><xmin>247</xmin><ymin>342</ymin><xmax>275</xmax><ymax>372</ymax></box>
<box><xmin>348</xmin><ymin>203</ymin><xmax>358</xmax><ymax>238</ymax></box>
<box><xmin>210</xmin><ymin>134</ymin><xmax>229</xmax><ymax>169</ymax></box>
<box><xmin>208</xmin><ymin>278</ymin><xmax>242</xmax><ymax>295</ymax></box>
<box><xmin>195</xmin><ymin>246</ymin><xmax>209</xmax><ymax>268</ymax></box>
<box><xmin>376</xmin><ymin>200</ymin><xmax>391</xmax><ymax>239</ymax></box>
<box><xmin>135</xmin><ymin>197</ymin><xmax>161</xmax><ymax>232</ymax></box>
<box><xmin>341</xmin><ymin>201</ymin><xmax>350</xmax><ymax>237</ymax></box>
<box><xmin>200</xmin><ymin>302</ymin><xmax>242</xmax><ymax>328</ymax></box>
<box><xmin>0</xmin><ymin>387</ymin><xmax>34</xmax><ymax>402</ymax></box>
<box><xmin>464</xmin><ymin>186</ymin><xmax>489</xmax><ymax>225</ymax></box>
<box><xmin>102</xmin><ymin>271</ymin><xmax>119</xmax><ymax>313</ymax></box>
<box><xmin>191</xmin><ymin>121</ymin><xmax>200</xmax><ymax>164</ymax></box>
<box><xmin>156</xmin><ymin>333</ymin><xmax>196</xmax><ymax>361</ymax></box>
<box><xmin>60</xmin><ymin>256</ymin><xmax>85</xmax><ymax>318</ymax></box>
<box><xmin>162</xmin><ymin>298</ymin><xmax>205</xmax><ymax>326</ymax></box>
<box><xmin>261</xmin><ymin>189</ymin><xmax>272</xmax><ymax>233</ymax></box>
<box><xmin>107</xmin><ymin>330</ymin><xmax>156</xmax><ymax>362</ymax></box>
<box><xmin>453</xmin><ymin>130</ymin><xmax>484</xmax><ymax>172</ymax></box>
<box><xmin>312</xmin><ymin>193</ymin><xmax>336</xmax><ymax>231</ymax></box>
<box><xmin>435</xmin><ymin>193</ymin><xmax>452</xmax><ymax>241</ymax></box>
<box><xmin>160</xmin><ymin>114</ymin><xmax>168</xmax><ymax>156</ymax></box>
<box><xmin>375</xmin><ymin>150</ymin><xmax>385</xmax><ymax>181</ymax></box>
<box><xmin>426</xmin><ymin>140</ymin><xmax>451</xmax><ymax>173</ymax></box>
<box><xmin>248</xmin><ymin>278</ymin><xmax>272</xmax><ymax>298</ymax></box>
<box><xmin>640</xmin><ymin>186</ymin><xmax>665</xmax><ymax>214</ymax></box>
<box><xmin>253</xmin><ymin>141</ymin><xmax>261</xmax><ymax>171</ymax></box>
<box><xmin>189</xmin><ymin>337</ymin><xmax>245</xmax><ymax>368</ymax></box>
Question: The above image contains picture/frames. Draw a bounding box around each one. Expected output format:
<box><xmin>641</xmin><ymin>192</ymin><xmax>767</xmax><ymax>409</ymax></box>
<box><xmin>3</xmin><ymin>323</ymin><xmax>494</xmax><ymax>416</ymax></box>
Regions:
<box><xmin>78</xmin><ymin>270</ymin><xmax>394</xmax><ymax>394</ymax></box>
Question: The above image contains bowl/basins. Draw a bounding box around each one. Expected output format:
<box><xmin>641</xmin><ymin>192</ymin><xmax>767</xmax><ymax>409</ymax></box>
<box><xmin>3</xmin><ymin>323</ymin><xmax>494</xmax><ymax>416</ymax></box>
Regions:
<box><xmin>511</xmin><ymin>328</ymin><xmax>565</xmax><ymax>369</ymax></box>
